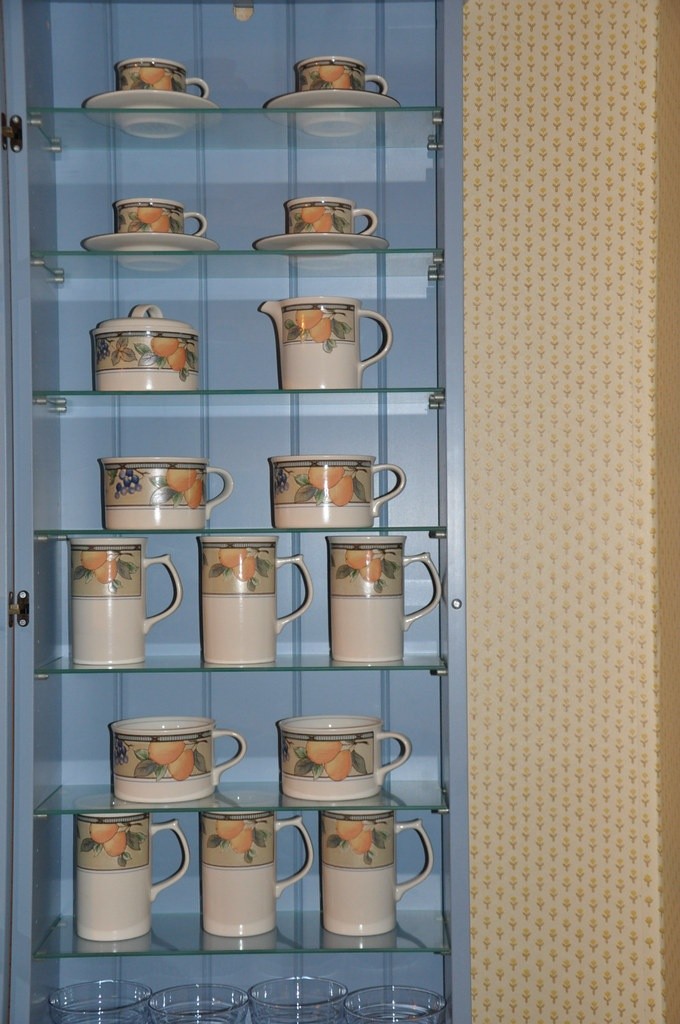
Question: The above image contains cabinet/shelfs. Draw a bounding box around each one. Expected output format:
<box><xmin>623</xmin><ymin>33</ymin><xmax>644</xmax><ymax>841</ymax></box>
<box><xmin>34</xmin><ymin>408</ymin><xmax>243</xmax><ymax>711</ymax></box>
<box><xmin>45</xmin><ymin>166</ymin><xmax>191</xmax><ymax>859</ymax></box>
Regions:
<box><xmin>32</xmin><ymin>105</ymin><xmax>453</xmax><ymax>959</ymax></box>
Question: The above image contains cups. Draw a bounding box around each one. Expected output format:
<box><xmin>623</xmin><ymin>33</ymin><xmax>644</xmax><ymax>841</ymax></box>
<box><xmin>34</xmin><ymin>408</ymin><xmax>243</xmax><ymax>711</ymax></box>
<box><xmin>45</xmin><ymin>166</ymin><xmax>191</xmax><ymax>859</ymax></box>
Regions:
<box><xmin>284</xmin><ymin>196</ymin><xmax>378</xmax><ymax>236</ymax></box>
<box><xmin>271</xmin><ymin>455</ymin><xmax>406</xmax><ymax>529</ymax></box>
<box><xmin>277</xmin><ymin>713</ymin><xmax>412</xmax><ymax>802</ymax></box>
<box><xmin>318</xmin><ymin>809</ymin><xmax>434</xmax><ymax>936</ymax></box>
<box><xmin>110</xmin><ymin>717</ymin><xmax>248</xmax><ymax>804</ymax></box>
<box><xmin>117</xmin><ymin>57</ymin><xmax>209</xmax><ymax>100</ymax></box>
<box><xmin>261</xmin><ymin>297</ymin><xmax>392</xmax><ymax>390</ymax></box>
<box><xmin>326</xmin><ymin>534</ymin><xmax>441</xmax><ymax>662</ymax></box>
<box><xmin>74</xmin><ymin>813</ymin><xmax>191</xmax><ymax>943</ymax></box>
<box><xmin>200</xmin><ymin>810</ymin><xmax>314</xmax><ymax>938</ymax></box>
<box><xmin>71</xmin><ymin>537</ymin><xmax>184</xmax><ymax>665</ymax></box>
<box><xmin>295</xmin><ymin>55</ymin><xmax>388</xmax><ymax>96</ymax></box>
<box><xmin>198</xmin><ymin>536</ymin><xmax>313</xmax><ymax>663</ymax></box>
<box><xmin>115</xmin><ymin>198</ymin><xmax>207</xmax><ymax>236</ymax></box>
<box><xmin>100</xmin><ymin>457</ymin><xmax>234</xmax><ymax>531</ymax></box>
<box><xmin>93</xmin><ymin>304</ymin><xmax>200</xmax><ymax>391</ymax></box>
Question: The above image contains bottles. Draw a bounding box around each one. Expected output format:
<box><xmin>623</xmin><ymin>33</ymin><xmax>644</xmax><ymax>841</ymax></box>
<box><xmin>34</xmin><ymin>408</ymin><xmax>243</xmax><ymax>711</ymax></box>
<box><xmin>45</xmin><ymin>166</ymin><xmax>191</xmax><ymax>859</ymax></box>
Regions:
<box><xmin>49</xmin><ymin>977</ymin><xmax>448</xmax><ymax>1024</ymax></box>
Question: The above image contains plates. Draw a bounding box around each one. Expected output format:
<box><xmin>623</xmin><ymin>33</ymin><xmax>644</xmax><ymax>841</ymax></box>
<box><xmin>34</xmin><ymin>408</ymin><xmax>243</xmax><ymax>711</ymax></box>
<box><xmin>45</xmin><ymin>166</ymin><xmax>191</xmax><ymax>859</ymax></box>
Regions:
<box><xmin>85</xmin><ymin>89</ymin><xmax>220</xmax><ymax>139</ymax></box>
<box><xmin>83</xmin><ymin>232</ymin><xmax>218</xmax><ymax>251</ymax></box>
<box><xmin>255</xmin><ymin>232</ymin><xmax>388</xmax><ymax>251</ymax></box>
<box><xmin>265</xmin><ymin>89</ymin><xmax>400</xmax><ymax>136</ymax></box>
